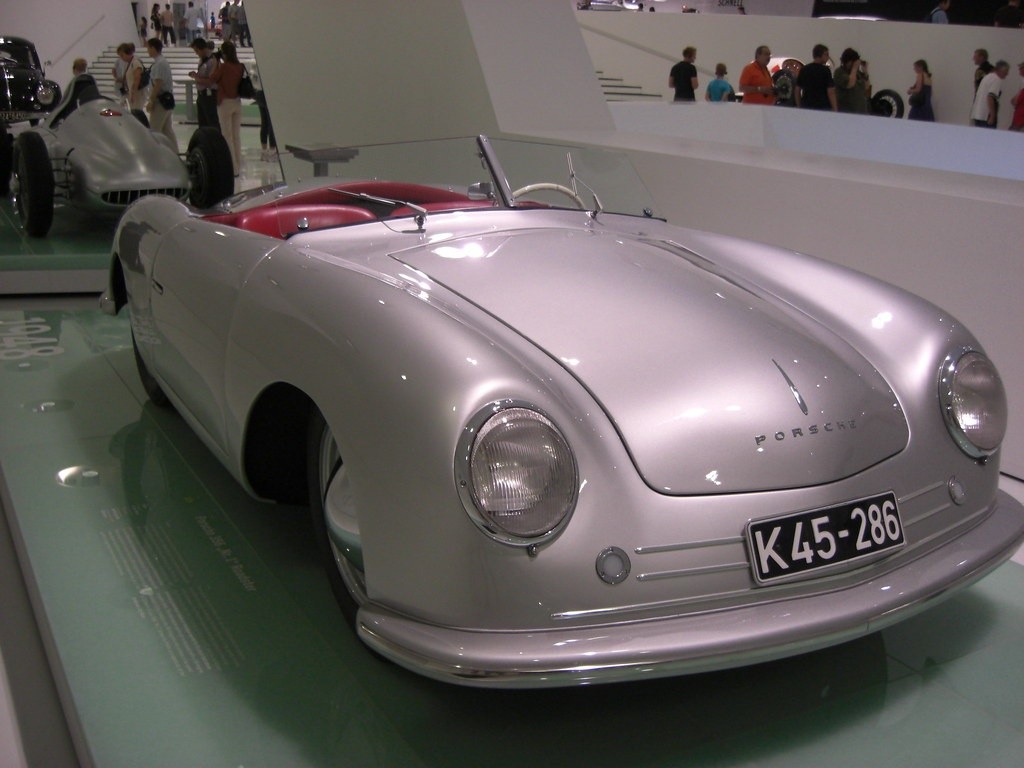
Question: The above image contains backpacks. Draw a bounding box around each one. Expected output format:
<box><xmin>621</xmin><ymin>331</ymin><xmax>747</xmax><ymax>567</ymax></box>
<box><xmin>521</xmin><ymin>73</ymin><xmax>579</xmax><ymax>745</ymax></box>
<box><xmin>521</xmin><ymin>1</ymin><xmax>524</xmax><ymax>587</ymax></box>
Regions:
<box><xmin>923</xmin><ymin>8</ymin><xmax>942</xmax><ymax>23</ymax></box>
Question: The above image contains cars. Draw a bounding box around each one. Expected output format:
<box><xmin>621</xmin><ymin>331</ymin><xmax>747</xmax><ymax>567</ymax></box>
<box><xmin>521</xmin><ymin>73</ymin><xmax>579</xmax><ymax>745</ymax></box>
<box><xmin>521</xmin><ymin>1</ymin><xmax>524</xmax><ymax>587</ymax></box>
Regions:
<box><xmin>16</xmin><ymin>73</ymin><xmax>236</xmax><ymax>238</ymax></box>
<box><xmin>589</xmin><ymin>0</ymin><xmax>644</xmax><ymax>11</ymax></box>
<box><xmin>0</xmin><ymin>33</ymin><xmax>63</xmax><ymax>113</ymax></box>
<box><xmin>96</xmin><ymin>134</ymin><xmax>1024</xmax><ymax>689</ymax></box>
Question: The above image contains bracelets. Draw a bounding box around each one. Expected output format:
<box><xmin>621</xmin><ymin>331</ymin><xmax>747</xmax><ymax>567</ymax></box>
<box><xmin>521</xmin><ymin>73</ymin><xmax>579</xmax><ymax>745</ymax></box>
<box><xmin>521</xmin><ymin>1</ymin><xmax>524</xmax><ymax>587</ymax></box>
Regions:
<box><xmin>758</xmin><ymin>86</ymin><xmax>760</xmax><ymax>92</ymax></box>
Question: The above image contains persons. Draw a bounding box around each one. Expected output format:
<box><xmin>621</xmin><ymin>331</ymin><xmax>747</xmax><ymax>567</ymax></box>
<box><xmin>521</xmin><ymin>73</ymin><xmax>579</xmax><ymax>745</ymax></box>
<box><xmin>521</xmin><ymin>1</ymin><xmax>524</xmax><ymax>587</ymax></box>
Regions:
<box><xmin>794</xmin><ymin>44</ymin><xmax>871</xmax><ymax>115</ymax></box>
<box><xmin>705</xmin><ymin>64</ymin><xmax>731</xmax><ymax>102</ymax></box>
<box><xmin>72</xmin><ymin>37</ymin><xmax>280</xmax><ymax>177</ymax></box>
<box><xmin>907</xmin><ymin>59</ymin><xmax>935</xmax><ymax>122</ymax></box>
<box><xmin>923</xmin><ymin>0</ymin><xmax>950</xmax><ymax>24</ymax></box>
<box><xmin>682</xmin><ymin>4</ymin><xmax>700</xmax><ymax>14</ymax></box>
<box><xmin>139</xmin><ymin>0</ymin><xmax>253</xmax><ymax>48</ymax></box>
<box><xmin>637</xmin><ymin>3</ymin><xmax>656</xmax><ymax>13</ymax></box>
<box><xmin>993</xmin><ymin>0</ymin><xmax>1024</xmax><ymax>29</ymax></box>
<box><xmin>737</xmin><ymin>6</ymin><xmax>747</xmax><ymax>15</ymax></box>
<box><xmin>738</xmin><ymin>46</ymin><xmax>776</xmax><ymax>105</ymax></box>
<box><xmin>969</xmin><ymin>48</ymin><xmax>1024</xmax><ymax>134</ymax></box>
<box><xmin>576</xmin><ymin>0</ymin><xmax>591</xmax><ymax>10</ymax></box>
<box><xmin>669</xmin><ymin>46</ymin><xmax>699</xmax><ymax>102</ymax></box>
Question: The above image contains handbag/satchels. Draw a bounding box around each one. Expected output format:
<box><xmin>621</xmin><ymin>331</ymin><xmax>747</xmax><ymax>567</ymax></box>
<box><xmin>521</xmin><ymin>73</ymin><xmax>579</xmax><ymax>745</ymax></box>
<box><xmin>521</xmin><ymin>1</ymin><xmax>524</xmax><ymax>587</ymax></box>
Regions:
<box><xmin>236</xmin><ymin>63</ymin><xmax>255</xmax><ymax>99</ymax></box>
<box><xmin>132</xmin><ymin>59</ymin><xmax>149</xmax><ymax>90</ymax></box>
<box><xmin>908</xmin><ymin>94</ymin><xmax>918</xmax><ymax>106</ymax></box>
<box><xmin>157</xmin><ymin>92</ymin><xmax>175</xmax><ymax>110</ymax></box>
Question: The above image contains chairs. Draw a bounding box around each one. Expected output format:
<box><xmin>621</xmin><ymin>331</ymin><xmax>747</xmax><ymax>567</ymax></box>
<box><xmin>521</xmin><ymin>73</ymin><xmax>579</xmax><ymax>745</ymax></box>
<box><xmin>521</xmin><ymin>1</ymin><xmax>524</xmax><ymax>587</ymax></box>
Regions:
<box><xmin>240</xmin><ymin>204</ymin><xmax>376</xmax><ymax>246</ymax></box>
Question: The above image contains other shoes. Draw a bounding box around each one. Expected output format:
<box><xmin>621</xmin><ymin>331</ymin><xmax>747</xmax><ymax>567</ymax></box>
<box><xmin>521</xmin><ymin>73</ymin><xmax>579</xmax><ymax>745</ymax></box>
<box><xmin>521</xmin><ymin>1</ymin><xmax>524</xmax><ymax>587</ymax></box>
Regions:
<box><xmin>260</xmin><ymin>150</ymin><xmax>279</xmax><ymax>162</ymax></box>
